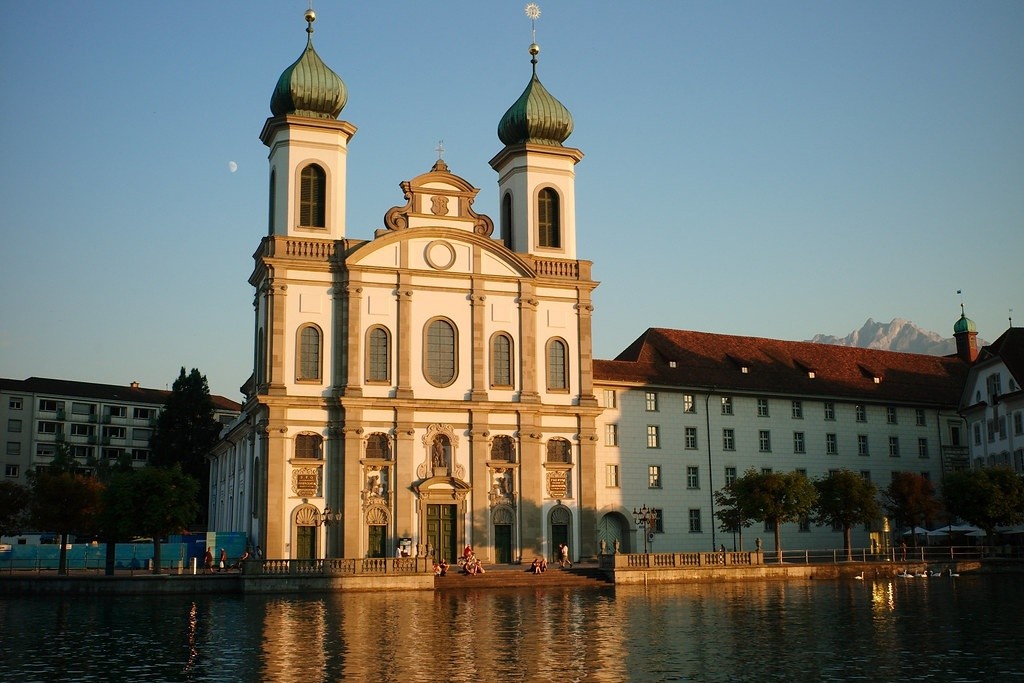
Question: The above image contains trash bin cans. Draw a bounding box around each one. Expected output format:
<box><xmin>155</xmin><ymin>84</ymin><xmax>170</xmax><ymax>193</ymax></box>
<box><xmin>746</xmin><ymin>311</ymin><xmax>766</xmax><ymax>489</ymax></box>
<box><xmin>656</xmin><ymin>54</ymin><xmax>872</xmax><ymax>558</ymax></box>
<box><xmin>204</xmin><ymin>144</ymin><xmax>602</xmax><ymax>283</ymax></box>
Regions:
<box><xmin>189</xmin><ymin>557</ymin><xmax>197</xmax><ymax>574</ymax></box>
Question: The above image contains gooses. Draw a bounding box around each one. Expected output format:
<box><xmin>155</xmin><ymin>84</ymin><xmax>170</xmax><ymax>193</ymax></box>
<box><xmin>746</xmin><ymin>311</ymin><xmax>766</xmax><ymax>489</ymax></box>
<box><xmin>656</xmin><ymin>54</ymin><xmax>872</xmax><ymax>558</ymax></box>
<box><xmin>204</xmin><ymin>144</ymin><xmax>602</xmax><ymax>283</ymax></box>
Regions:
<box><xmin>897</xmin><ymin>569</ymin><xmax>907</xmax><ymax>578</ymax></box>
<box><xmin>930</xmin><ymin>570</ymin><xmax>941</xmax><ymax>576</ymax></box>
<box><xmin>854</xmin><ymin>572</ymin><xmax>864</xmax><ymax>579</ymax></box>
<box><xmin>903</xmin><ymin>572</ymin><xmax>914</xmax><ymax>578</ymax></box>
<box><xmin>914</xmin><ymin>569</ymin><xmax>921</xmax><ymax>577</ymax></box>
<box><xmin>920</xmin><ymin>570</ymin><xmax>927</xmax><ymax>578</ymax></box>
<box><xmin>948</xmin><ymin>569</ymin><xmax>959</xmax><ymax>577</ymax></box>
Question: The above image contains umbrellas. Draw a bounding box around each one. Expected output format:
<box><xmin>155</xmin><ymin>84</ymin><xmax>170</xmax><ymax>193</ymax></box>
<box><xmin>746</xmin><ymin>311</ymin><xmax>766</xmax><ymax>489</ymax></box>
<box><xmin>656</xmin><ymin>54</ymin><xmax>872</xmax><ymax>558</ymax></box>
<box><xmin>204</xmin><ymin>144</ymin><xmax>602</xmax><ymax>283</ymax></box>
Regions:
<box><xmin>904</xmin><ymin>524</ymin><xmax>1024</xmax><ymax>536</ymax></box>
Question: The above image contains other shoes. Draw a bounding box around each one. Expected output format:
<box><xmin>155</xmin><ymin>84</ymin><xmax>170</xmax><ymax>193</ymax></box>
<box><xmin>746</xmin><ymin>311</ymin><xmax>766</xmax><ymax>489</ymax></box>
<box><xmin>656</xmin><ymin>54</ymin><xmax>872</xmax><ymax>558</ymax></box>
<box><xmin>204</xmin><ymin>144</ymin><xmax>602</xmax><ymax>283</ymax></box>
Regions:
<box><xmin>570</xmin><ymin>564</ymin><xmax>573</xmax><ymax>568</ymax></box>
<box><xmin>224</xmin><ymin>570</ymin><xmax>229</xmax><ymax>572</ymax></box>
<box><xmin>561</xmin><ymin>566</ymin><xmax>565</xmax><ymax>568</ymax></box>
<box><xmin>216</xmin><ymin>570</ymin><xmax>222</xmax><ymax>572</ymax></box>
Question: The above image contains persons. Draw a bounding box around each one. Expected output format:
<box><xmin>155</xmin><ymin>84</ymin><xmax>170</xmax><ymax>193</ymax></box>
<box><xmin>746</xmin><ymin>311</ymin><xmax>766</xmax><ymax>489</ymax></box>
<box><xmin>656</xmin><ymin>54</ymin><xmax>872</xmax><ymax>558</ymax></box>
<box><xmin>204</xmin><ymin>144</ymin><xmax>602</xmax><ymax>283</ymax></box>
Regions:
<box><xmin>431</xmin><ymin>544</ymin><xmax>481</xmax><ymax>575</ymax></box>
<box><xmin>531</xmin><ymin>558</ymin><xmax>548</xmax><ymax>573</ymax></box>
<box><xmin>204</xmin><ymin>547</ymin><xmax>214</xmax><ymax>573</ymax></box>
<box><xmin>721</xmin><ymin>543</ymin><xmax>725</xmax><ymax>551</ymax></box>
<box><xmin>395</xmin><ymin>544</ymin><xmax>409</xmax><ymax>557</ymax></box>
<box><xmin>558</xmin><ymin>543</ymin><xmax>572</xmax><ymax>568</ymax></box>
<box><xmin>242</xmin><ymin>546</ymin><xmax>262</xmax><ymax>560</ymax></box>
<box><xmin>900</xmin><ymin>541</ymin><xmax>907</xmax><ymax>561</ymax></box>
<box><xmin>216</xmin><ymin>548</ymin><xmax>228</xmax><ymax>572</ymax></box>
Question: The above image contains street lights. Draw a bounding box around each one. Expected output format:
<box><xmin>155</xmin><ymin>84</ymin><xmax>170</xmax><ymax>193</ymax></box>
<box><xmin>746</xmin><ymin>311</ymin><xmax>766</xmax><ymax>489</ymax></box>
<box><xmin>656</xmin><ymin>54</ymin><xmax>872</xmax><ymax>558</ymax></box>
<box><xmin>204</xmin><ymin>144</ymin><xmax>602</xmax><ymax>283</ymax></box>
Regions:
<box><xmin>632</xmin><ymin>503</ymin><xmax>656</xmax><ymax>554</ymax></box>
<box><xmin>313</xmin><ymin>504</ymin><xmax>342</xmax><ymax>559</ymax></box>
<box><xmin>736</xmin><ymin>513</ymin><xmax>742</xmax><ymax>551</ymax></box>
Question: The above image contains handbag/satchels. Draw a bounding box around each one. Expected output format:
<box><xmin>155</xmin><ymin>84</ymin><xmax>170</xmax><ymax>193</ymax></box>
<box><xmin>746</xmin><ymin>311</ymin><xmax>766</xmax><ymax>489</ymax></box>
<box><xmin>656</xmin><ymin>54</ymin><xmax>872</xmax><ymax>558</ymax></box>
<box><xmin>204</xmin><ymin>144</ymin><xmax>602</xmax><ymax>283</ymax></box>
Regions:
<box><xmin>220</xmin><ymin>561</ymin><xmax>224</xmax><ymax>568</ymax></box>
